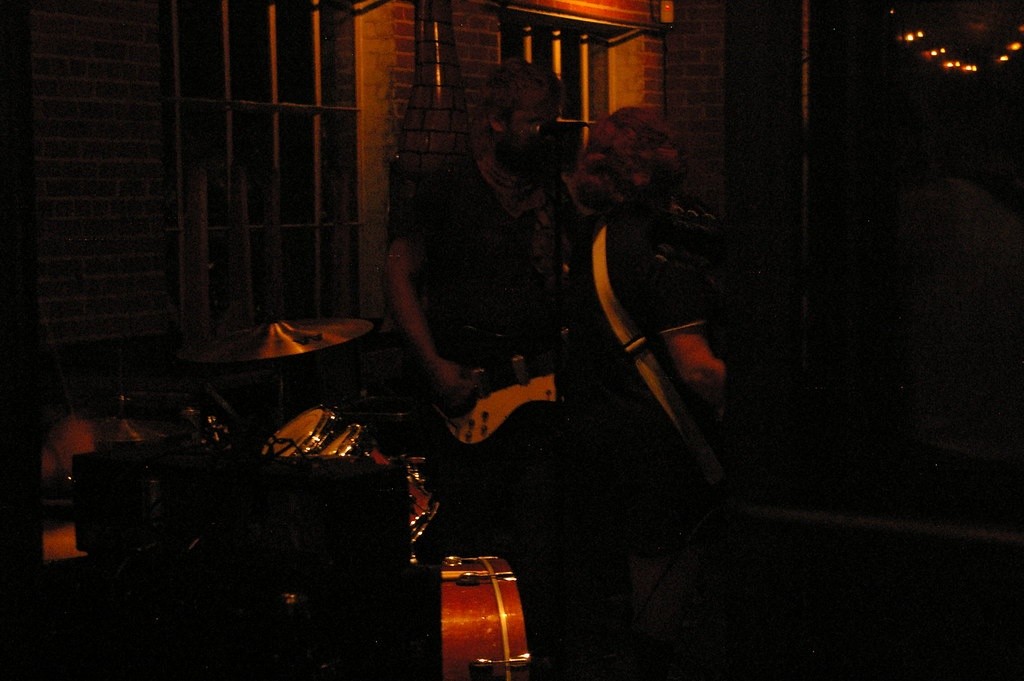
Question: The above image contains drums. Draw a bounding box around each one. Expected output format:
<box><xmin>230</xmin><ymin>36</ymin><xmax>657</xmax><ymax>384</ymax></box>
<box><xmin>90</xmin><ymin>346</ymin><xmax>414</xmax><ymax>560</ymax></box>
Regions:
<box><xmin>438</xmin><ymin>555</ymin><xmax>532</xmax><ymax>681</ymax></box>
<box><xmin>255</xmin><ymin>403</ymin><xmax>439</xmax><ymax>545</ymax></box>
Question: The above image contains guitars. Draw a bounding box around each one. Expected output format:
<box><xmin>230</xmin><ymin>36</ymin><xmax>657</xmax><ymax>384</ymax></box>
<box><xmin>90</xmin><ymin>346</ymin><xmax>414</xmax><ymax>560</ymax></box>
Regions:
<box><xmin>443</xmin><ymin>315</ymin><xmax>708</xmax><ymax>444</ymax></box>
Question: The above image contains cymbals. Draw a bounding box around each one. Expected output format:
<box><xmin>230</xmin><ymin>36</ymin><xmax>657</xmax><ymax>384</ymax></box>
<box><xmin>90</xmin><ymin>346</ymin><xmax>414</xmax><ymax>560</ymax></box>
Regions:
<box><xmin>91</xmin><ymin>420</ymin><xmax>168</xmax><ymax>442</ymax></box>
<box><xmin>167</xmin><ymin>307</ymin><xmax>380</xmax><ymax>367</ymax></box>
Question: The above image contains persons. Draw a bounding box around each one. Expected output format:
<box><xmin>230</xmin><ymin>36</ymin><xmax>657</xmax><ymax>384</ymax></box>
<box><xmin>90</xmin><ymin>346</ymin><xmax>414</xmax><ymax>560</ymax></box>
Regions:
<box><xmin>571</xmin><ymin>106</ymin><xmax>729</xmax><ymax>680</ymax></box>
<box><xmin>380</xmin><ymin>58</ymin><xmax>583</xmax><ymax>547</ymax></box>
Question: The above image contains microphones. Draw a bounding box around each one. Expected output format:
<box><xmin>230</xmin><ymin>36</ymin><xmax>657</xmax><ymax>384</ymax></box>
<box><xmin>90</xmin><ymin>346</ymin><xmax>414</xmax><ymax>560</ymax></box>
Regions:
<box><xmin>528</xmin><ymin>119</ymin><xmax>586</xmax><ymax>142</ymax></box>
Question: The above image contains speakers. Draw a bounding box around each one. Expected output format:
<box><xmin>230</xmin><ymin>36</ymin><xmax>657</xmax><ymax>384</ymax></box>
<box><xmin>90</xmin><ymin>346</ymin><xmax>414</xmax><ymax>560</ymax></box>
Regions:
<box><xmin>729</xmin><ymin>505</ymin><xmax>1024</xmax><ymax>681</ymax></box>
